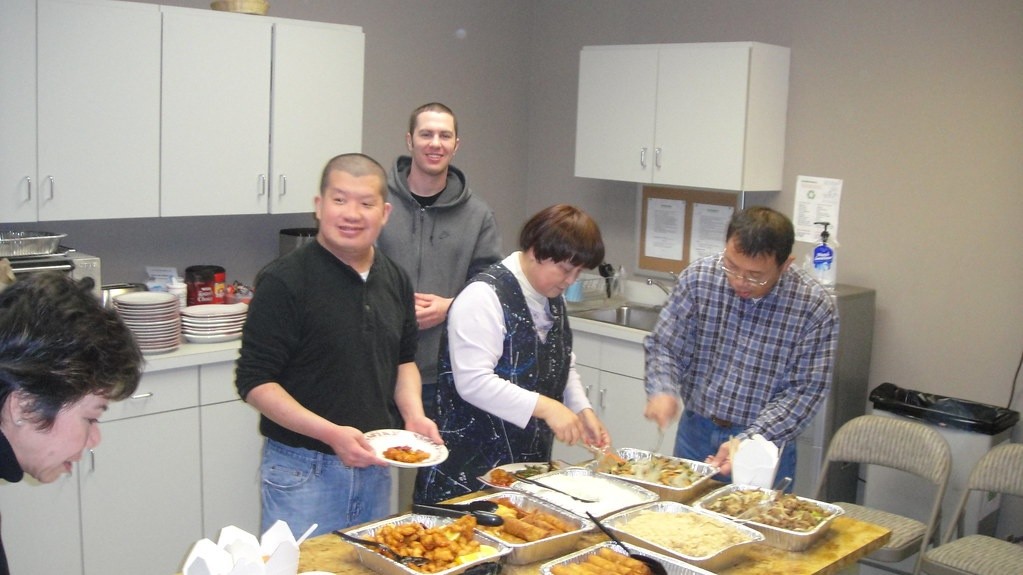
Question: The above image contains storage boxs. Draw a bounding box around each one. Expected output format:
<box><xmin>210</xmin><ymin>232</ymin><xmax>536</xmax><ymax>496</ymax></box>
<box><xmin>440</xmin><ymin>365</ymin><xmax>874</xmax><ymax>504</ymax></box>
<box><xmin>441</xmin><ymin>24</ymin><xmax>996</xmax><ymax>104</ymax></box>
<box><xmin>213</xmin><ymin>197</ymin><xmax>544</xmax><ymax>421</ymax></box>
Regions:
<box><xmin>587</xmin><ymin>446</ymin><xmax>720</xmax><ymax>504</ymax></box>
<box><xmin>539</xmin><ymin>539</ymin><xmax>717</xmax><ymax>575</ymax></box>
<box><xmin>343</xmin><ymin>513</ymin><xmax>514</xmax><ymax>575</ymax></box>
<box><xmin>599</xmin><ymin>500</ymin><xmax>766</xmax><ymax>572</ymax></box>
<box><xmin>510</xmin><ymin>466</ymin><xmax>662</xmax><ymax>533</ymax></box>
<box><xmin>447</xmin><ymin>490</ymin><xmax>596</xmax><ymax>565</ymax></box>
<box><xmin>693</xmin><ymin>482</ymin><xmax>847</xmax><ymax>552</ymax></box>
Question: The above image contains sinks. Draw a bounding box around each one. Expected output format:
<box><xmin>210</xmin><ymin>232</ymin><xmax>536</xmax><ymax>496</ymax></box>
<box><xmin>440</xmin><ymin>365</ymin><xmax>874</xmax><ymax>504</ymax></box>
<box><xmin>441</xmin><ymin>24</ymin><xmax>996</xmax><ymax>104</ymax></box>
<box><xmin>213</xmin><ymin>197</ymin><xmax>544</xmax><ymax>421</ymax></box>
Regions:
<box><xmin>566</xmin><ymin>302</ymin><xmax>663</xmax><ymax>332</ymax></box>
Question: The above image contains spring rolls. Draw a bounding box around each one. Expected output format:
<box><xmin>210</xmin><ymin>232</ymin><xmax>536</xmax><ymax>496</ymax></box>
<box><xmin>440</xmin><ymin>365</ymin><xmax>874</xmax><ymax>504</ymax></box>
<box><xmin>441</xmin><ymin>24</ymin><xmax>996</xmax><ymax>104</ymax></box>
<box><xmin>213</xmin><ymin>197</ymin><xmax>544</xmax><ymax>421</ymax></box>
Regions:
<box><xmin>497</xmin><ymin>512</ymin><xmax>578</xmax><ymax>545</ymax></box>
<box><xmin>550</xmin><ymin>547</ymin><xmax>653</xmax><ymax>575</ymax></box>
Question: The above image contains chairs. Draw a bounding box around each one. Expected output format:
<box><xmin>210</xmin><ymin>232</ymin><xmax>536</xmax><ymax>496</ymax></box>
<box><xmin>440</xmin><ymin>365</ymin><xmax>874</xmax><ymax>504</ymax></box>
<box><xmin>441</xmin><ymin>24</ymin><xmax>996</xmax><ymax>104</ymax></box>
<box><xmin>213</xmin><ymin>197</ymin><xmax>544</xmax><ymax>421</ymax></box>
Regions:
<box><xmin>813</xmin><ymin>413</ymin><xmax>952</xmax><ymax>575</ymax></box>
<box><xmin>918</xmin><ymin>438</ymin><xmax>1023</xmax><ymax>575</ymax></box>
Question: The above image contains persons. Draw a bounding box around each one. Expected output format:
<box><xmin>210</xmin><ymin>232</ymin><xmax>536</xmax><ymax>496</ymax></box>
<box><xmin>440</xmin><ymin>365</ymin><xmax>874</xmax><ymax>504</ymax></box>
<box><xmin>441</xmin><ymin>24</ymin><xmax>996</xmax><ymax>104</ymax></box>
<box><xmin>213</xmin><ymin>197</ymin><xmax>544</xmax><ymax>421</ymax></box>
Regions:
<box><xmin>376</xmin><ymin>103</ymin><xmax>508</xmax><ymax>516</ymax></box>
<box><xmin>411</xmin><ymin>204</ymin><xmax>611</xmax><ymax>506</ymax></box>
<box><xmin>0</xmin><ymin>270</ymin><xmax>146</xmax><ymax>575</ymax></box>
<box><xmin>643</xmin><ymin>205</ymin><xmax>839</xmax><ymax>494</ymax></box>
<box><xmin>236</xmin><ymin>152</ymin><xmax>445</xmax><ymax>542</ymax></box>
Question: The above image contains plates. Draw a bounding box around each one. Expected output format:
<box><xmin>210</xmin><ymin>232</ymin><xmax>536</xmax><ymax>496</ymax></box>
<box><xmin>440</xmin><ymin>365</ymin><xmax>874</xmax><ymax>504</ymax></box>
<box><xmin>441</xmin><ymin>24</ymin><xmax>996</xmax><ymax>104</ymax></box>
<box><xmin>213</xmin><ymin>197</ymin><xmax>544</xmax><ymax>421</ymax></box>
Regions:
<box><xmin>179</xmin><ymin>302</ymin><xmax>249</xmax><ymax>343</ymax></box>
<box><xmin>478</xmin><ymin>462</ymin><xmax>553</xmax><ymax>491</ymax></box>
<box><xmin>363</xmin><ymin>428</ymin><xmax>449</xmax><ymax>469</ymax></box>
<box><xmin>111</xmin><ymin>291</ymin><xmax>182</xmax><ymax>356</ymax></box>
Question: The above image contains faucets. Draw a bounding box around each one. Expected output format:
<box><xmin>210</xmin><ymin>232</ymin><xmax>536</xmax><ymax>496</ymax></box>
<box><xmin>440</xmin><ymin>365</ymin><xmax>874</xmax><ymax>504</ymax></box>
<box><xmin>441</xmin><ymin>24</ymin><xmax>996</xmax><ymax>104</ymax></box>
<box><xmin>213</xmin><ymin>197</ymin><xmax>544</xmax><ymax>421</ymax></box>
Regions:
<box><xmin>646</xmin><ymin>277</ymin><xmax>671</xmax><ymax>297</ymax></box>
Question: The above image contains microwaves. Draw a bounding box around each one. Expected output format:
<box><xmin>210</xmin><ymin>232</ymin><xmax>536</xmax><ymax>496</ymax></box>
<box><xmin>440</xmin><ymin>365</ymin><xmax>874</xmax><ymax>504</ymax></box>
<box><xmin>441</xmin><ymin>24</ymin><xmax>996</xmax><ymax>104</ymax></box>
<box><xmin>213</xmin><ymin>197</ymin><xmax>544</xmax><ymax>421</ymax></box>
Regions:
<box><xmin>0</xmin><ymin>250</ymin><xmax>102</xmax><ymax>299</ymax></box>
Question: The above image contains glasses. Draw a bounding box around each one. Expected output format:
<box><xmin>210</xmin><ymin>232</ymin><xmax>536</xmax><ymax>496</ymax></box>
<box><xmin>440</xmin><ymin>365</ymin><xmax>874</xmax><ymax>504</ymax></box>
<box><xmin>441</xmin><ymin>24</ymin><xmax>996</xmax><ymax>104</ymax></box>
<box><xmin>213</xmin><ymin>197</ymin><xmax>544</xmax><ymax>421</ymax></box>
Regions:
<box><xmin>719</xmin><ymin>248</ymin><xmax>780</xmax><ymax>286</ymax></box>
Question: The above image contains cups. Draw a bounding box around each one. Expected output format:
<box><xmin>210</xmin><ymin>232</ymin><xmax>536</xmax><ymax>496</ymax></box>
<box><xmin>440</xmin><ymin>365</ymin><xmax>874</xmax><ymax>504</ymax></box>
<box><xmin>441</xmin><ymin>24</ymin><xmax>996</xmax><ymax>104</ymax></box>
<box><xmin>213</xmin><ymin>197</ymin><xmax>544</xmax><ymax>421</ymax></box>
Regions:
<box><xmin>185</xmin><ymin>264</ymin><xmax>228</xmax><ymax>307</ymax></box>
<box><xmin>166</xmin><ymin>282</ymin><xmax>188</xmax><ymax>308</ymax></box>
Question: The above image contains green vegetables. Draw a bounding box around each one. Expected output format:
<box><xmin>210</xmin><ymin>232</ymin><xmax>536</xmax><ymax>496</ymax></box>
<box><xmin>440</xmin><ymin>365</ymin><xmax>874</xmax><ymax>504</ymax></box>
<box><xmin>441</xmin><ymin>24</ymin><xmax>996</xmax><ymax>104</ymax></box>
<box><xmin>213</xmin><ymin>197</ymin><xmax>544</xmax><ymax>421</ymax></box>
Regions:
<box><xmin>513</xmin><ymin>464</ymin><xmax>543</xmax><ymax>478</ymax></box>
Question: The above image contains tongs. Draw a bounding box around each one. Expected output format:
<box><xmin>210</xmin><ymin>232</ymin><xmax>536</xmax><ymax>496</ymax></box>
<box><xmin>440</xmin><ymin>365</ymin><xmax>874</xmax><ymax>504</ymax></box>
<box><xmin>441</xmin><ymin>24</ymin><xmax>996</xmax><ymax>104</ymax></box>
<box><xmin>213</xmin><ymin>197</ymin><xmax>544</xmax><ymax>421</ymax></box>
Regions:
<box><xmin>412</xmin><ymin>501</ymin><xmax>505</xmax><ymax>527</ymax></box>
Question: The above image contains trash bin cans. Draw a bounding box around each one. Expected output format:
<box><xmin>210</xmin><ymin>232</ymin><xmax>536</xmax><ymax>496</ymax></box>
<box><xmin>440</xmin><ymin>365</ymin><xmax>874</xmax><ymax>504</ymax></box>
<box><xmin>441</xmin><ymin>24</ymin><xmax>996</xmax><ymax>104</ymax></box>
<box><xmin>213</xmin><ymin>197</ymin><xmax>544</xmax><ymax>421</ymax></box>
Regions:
<box><xmin>856</xmin><ymin>381</ymin><xmax>1020</xmax><ymax>571</ymax></box>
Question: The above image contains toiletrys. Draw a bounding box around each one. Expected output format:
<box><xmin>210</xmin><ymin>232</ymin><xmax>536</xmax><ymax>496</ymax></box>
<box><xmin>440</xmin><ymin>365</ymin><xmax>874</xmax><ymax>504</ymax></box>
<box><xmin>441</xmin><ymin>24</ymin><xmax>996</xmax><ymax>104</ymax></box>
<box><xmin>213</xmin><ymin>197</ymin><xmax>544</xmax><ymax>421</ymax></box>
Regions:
<box><xmin>802</xmin><ymin>221</ymin><xmax>839</xmax><ymax>295</ymax></box>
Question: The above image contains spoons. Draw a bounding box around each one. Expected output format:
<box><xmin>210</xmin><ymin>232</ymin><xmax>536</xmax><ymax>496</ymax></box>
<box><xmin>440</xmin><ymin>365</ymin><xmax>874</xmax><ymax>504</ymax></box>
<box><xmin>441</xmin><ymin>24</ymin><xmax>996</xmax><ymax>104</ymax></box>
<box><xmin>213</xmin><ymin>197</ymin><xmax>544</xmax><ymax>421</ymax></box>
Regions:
<box><xmin>331</xmin><ymin>530</ymin><xmax>430</xmax><ymax>566</ymax></box>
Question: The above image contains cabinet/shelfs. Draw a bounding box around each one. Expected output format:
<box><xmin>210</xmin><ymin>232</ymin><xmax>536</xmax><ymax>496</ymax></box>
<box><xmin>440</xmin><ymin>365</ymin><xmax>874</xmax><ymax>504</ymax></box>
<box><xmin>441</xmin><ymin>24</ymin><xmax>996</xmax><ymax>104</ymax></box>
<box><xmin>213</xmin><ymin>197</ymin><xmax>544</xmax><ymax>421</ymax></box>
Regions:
<box><xmin>0</xmin><ymin>0</ymin><xmax>366</xmax><ymax>225</ymax></box>
<box><xmin>573</xmin><ymin>38</ymin><xmax>796</xmax><ymax>194</ymax></box>
<box><xmin>0</xmin><ymin>316</ymin><xmax>684</xmax><ymax>575</ymax></box>
